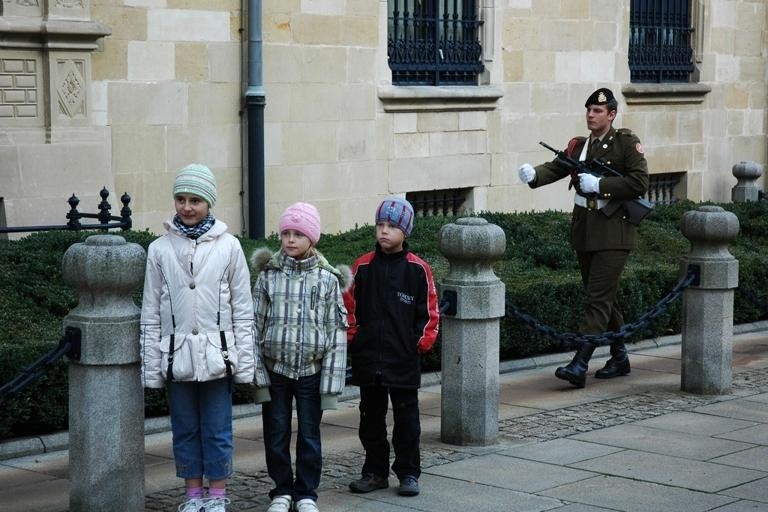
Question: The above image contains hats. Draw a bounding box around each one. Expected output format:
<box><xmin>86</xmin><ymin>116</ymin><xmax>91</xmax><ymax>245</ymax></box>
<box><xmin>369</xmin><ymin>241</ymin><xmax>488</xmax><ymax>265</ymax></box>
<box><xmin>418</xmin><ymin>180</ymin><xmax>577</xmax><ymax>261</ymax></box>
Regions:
<box><xmin>171</xmin><ymin>162</ymin><xmax>218</xmax><ymax>209</ymax></box>
<box><xmin>584</xmin><ymin>87</ymin><xmax>613</xmax><ymax>108</ymax></box>
<box><xmin>276</xmin><ymin>200</ymin><xmax>322</xmax><ymax>246</ymax></box>
<box><xmin>374</xmin><ymin>194</ymin><xmax>415</xmax><ymax>237</ymax></box>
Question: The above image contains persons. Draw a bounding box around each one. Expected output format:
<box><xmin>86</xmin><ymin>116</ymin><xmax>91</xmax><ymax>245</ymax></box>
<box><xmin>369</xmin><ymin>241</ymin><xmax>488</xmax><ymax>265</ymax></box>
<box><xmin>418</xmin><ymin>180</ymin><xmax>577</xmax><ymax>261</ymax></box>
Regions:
<box><xmin>140</xmin><ymin>164</ymin><xmax>256</xmax><ymax>510</ymax></box>
<box><xmin>341</xmin><ymin>195</ymin><xmax>441</xmax><ymax>496</ymax></box>
<box><xmin>519</xmin><ymin>88</ymin><xmax>649</xmax><ymax>388</ymax></box>
<box><xmin>251</xmin><ymin>202</ymin><xmax>350</xmax><ymax>512</ymax></box>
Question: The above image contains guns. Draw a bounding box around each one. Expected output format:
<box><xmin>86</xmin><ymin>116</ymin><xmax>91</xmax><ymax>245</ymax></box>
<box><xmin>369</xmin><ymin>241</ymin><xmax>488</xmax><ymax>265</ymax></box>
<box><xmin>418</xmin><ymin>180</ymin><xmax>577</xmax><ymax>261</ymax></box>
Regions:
<box><xmin>539</xmin><ymin>141</ymin><xmax>654</xmax><ymax>226</ymax></box>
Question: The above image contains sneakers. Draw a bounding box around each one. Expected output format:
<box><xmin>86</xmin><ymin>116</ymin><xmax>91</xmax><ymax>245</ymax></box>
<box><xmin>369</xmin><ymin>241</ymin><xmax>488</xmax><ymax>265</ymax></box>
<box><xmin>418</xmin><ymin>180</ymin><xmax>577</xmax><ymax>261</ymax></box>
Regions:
<box><xmin>292</xmin><ymin>496</ymin><xmax>320</xmax><ymax>512</ymax></box>
<box><xmin>178</xmin><ymin>497</ymin><xmax>203</xmax><ymax>512</ymax></box>
<box><xmin>203</xmin><ymin>496</ymin><xmax>231</xmax><ymax>512</ymax></box>
<box><xmin>397</xmin><ymin>474</ymin><xmax>421</xmax><ymax>497</ymax></box>
<box><xmin>266</xmin><ymin>494</ymin><xmax>292</xmax><ymax>512</ymax></box>
<box><xmin>348</xmin><ymin>471</ymin><xmax>391</xmax><ymax>492</ymax></box>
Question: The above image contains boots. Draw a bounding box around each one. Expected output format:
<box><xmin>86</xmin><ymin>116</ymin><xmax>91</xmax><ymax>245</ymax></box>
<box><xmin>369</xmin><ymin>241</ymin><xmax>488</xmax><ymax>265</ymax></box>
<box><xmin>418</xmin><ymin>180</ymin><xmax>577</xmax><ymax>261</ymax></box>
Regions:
<box><xmin>594</xmin><ymin>339</ymin><xmax>632</xmax><ymax>380</ymax></box>
<box><xmin>554</xmin><ymin>342</ymin><xmax>597</xmax><ymax>389</ymax></box>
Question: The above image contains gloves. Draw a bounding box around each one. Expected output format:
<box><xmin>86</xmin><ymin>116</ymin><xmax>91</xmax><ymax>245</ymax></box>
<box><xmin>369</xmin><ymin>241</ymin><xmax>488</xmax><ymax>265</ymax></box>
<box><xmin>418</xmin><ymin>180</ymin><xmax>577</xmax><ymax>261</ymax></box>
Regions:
<box><xmin>517</xmin><ymin>163</ymin><xmax>537</xmax><ymax>184</ymax></box>
<box><xmin>576</xmin><ymin>172</ymin><xmax>602</xmax><ymax>196</ymax></box>
